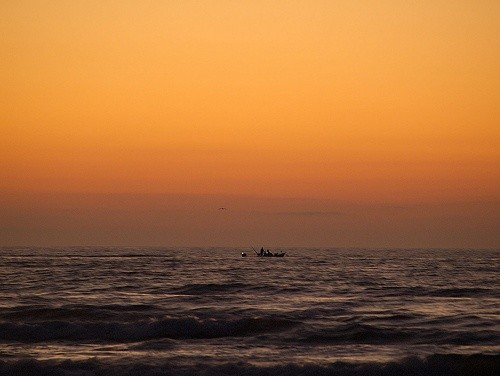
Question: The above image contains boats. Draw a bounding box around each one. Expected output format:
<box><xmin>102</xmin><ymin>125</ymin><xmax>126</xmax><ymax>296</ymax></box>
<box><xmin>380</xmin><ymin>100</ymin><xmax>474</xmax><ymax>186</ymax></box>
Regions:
<box><xmin>251</xmin><ymin>247</ymin><xmax>286</xmax><ymax>258</ymax></box>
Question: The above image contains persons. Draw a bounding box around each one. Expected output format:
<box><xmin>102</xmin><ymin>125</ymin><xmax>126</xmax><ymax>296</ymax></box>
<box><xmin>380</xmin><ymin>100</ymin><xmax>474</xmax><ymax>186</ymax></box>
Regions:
<box><xmin>261</xmin><ymin>248</ymin><xmax>264</xmax><ymax>253</ymax></box>
